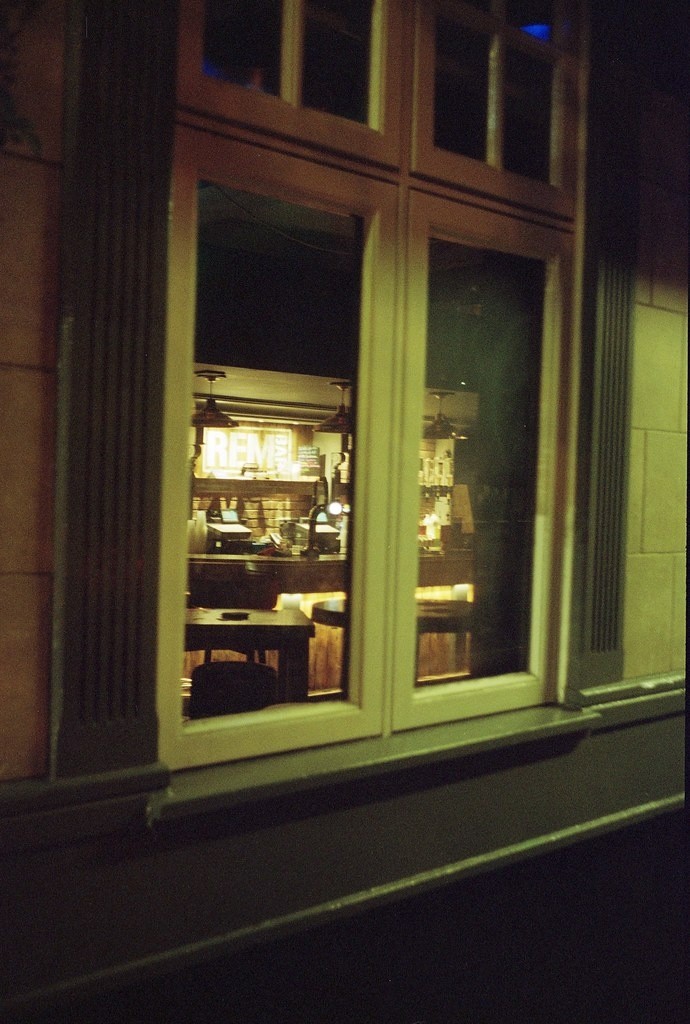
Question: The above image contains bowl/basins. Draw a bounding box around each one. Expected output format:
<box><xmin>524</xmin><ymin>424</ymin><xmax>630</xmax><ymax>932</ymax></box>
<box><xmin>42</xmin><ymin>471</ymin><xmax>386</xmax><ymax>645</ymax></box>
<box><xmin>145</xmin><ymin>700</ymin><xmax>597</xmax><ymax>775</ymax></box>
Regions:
<box><xmin>222</xmin><ymin>612</ymin><xmax>249</xmax><ymax>620</ymax></box>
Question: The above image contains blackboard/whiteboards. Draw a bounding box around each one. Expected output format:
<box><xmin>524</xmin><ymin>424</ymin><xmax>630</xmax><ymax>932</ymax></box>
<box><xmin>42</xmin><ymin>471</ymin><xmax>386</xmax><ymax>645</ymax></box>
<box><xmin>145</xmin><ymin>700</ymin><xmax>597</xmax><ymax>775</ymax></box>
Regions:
<box><xmin>297</xmin><ymin>446</ymin><xmax>319</xmax><ymax>477</ymax></box>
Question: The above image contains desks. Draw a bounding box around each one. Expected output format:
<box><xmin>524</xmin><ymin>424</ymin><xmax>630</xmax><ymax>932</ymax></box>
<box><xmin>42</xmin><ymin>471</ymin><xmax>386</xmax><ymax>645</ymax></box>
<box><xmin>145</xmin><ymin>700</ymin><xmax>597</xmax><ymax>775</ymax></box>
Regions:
<box><xmin>311</xmin><ymin>600</ymin><xmax>473</xmax><ymax>635</ymax></box>
<box><xmin>185</xmin><ymin>609</ymin><xmax>315</xmax><ymax>705</ymax></box>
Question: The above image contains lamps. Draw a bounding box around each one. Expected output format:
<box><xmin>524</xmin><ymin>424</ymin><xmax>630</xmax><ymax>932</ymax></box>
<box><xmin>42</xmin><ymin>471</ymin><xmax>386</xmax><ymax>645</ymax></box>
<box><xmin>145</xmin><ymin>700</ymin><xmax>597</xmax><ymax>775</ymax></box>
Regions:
<box><xmin>191</xmin><ymin>374</ymin><xmax>240</xmax><ymax>428</ymax></box>
<box><xmin>423</xmin><ymin>392</ymin><xmax>469</xmax><ymax>439</ymax></box>
<box><xmin>312</xmin><ymin>382</ymin><xmax>353</xmax><ymax>433</ymax></box>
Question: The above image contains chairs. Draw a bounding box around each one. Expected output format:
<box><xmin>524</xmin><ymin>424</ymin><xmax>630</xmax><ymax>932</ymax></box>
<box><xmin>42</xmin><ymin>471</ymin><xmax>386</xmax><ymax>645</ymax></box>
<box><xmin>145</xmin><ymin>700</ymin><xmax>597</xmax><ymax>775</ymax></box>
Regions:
<box><xmin>187</xmin><ymin>574</ymin><xmax>277</xmax><ymax>719</ymax></box>
